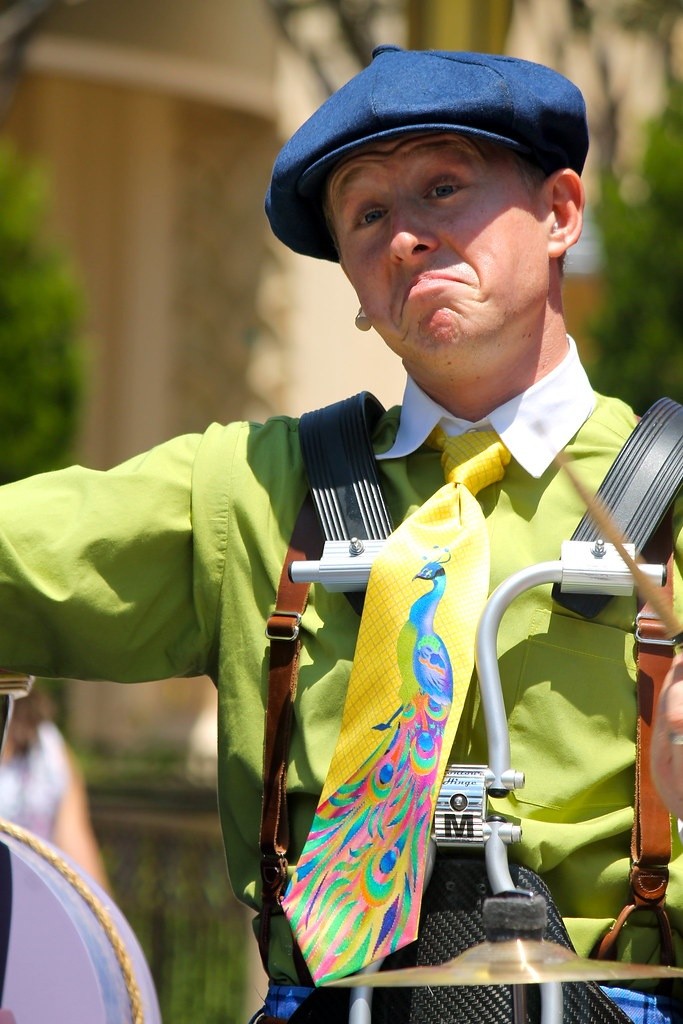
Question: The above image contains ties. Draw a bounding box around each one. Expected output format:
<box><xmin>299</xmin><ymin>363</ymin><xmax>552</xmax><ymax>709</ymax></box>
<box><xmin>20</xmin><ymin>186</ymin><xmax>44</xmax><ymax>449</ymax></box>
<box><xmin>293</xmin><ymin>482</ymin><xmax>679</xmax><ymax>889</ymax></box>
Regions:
<box><xmin>281</xmin><ymin>423</ymin><xmax>512</xmax><ymax>988</ymax></box>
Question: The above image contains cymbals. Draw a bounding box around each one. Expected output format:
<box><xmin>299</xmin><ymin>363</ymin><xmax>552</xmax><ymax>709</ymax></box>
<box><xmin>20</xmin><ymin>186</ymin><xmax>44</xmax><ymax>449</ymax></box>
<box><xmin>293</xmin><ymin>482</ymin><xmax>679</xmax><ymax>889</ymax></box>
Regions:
<box><xmin>322</xmin><ymin>941</ymin><xmax>683</xmax><ymax>990</ymax></box>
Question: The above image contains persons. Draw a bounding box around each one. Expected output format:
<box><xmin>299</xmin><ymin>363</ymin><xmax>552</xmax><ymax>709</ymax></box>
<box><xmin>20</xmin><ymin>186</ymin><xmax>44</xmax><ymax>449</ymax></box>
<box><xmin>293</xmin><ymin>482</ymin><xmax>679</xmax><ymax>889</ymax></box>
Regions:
<box><xmin>1</xmin><ymin>45</ymin><xmax>683</xmax><ymax>1023</ymax></box>
<box><xmin>2</xmin><ymin>686</ymin><xmax>110</xmax><ymax>894</ymax></box>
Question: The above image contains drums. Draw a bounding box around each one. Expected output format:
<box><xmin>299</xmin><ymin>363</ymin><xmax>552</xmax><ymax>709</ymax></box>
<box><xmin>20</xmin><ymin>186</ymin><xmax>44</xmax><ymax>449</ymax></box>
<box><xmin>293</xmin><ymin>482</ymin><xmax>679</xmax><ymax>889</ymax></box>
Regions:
<box><xmin>0</xmin><ymin>819</ymin><xmax>160</xmax><ymax>1024</ymax></box>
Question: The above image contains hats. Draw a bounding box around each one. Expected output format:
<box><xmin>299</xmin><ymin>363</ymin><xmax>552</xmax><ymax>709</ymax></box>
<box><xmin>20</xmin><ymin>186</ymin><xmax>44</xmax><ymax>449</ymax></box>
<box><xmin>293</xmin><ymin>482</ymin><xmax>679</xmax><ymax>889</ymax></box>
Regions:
<box><xmin>264</xmin><ymin>45</ymin><xmax>589</xmax><ymax>261</ymax></box>
